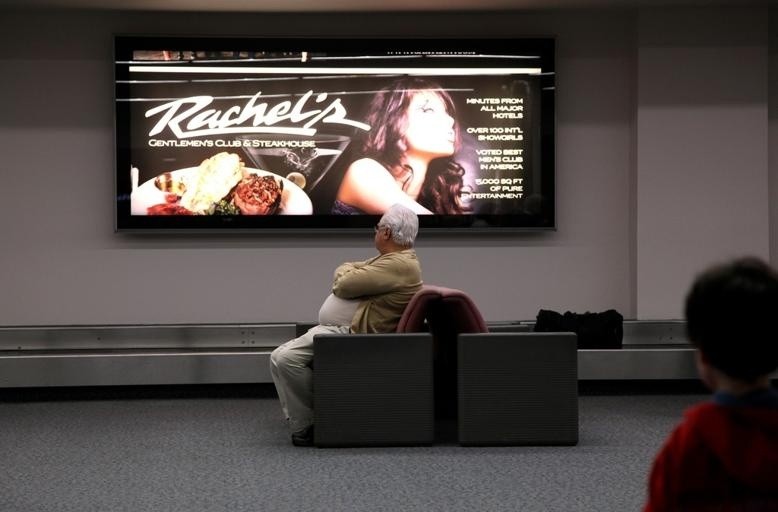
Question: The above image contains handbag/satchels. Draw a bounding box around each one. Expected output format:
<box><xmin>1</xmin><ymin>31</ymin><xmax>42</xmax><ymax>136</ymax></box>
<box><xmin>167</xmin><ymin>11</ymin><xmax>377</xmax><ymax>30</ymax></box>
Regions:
<box><xmin>533</xmin><ymin>307</ymin><xmax>625</xmax><ymax>350</ymax></box>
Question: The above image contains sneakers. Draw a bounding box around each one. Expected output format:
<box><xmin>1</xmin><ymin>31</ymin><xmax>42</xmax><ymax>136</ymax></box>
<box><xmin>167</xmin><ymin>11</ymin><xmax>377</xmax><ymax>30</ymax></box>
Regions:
<box><xmin>290</xmin><ymin>426</ymin><xmax>316</xmax><ymax>446</ymax></box>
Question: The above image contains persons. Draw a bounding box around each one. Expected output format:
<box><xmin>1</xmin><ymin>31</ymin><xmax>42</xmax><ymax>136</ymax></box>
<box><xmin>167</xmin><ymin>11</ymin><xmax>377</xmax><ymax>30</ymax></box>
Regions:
<box><xmin>269</xmin><ymin>205</ymin><xmax>425</xmax><ymax>447</ymax></box>
<box><xmin>329</xmin><ymin>76</ymin><xmax>465</xmax><ymax>216</ymax></box>
<box><xmin>643</xmin><ymin>254</ymin><xmax>777</xmax><ymax>510</ymax></box>
